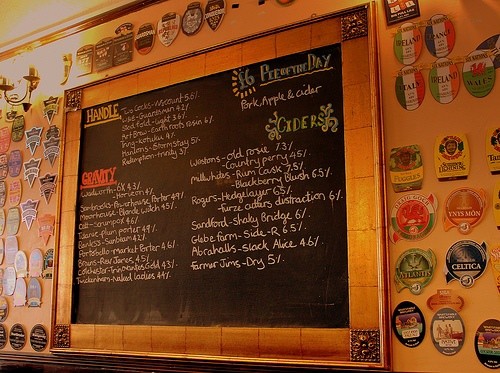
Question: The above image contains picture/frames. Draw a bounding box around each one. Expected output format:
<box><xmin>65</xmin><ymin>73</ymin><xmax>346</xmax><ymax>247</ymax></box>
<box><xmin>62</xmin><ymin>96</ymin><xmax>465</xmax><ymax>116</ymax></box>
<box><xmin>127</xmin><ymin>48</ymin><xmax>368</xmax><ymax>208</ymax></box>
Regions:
<box><xmin>48</xmin><ymin>0</ymin><xmax>394</xmax><ymax>372</ymax></box>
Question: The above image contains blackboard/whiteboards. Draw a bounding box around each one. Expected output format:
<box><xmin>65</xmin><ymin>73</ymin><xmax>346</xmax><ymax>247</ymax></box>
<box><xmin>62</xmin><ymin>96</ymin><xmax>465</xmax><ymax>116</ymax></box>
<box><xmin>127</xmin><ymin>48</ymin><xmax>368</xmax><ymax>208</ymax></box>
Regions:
<box><xmin>64</xmin><ymin>41</ymin><xmax>355</xmax><ymax>330</ymax></box>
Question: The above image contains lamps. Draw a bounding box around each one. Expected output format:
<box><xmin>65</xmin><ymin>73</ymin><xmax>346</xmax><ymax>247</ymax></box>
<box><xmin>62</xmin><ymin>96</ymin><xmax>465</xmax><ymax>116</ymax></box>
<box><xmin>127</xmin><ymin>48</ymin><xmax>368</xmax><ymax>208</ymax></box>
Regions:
<box><xmin>0</xmin><ymin>65</ymin><xmax>42</xmax><ymax>108</ymax></box>
